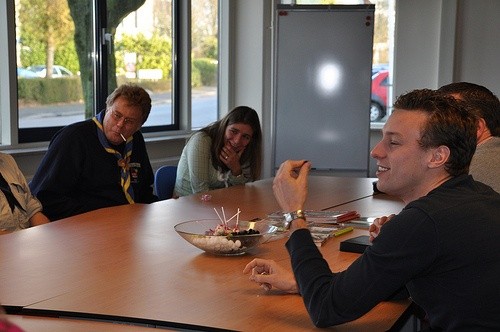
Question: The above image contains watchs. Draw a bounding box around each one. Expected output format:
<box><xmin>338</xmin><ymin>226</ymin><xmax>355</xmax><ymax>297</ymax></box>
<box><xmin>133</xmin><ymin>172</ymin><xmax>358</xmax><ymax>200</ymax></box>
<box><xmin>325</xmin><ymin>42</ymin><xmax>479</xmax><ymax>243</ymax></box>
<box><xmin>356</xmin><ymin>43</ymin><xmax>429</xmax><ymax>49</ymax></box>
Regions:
<box><xmin>280</xmin><ymin>210</ymin><xmax>306</xmax><ymax>230</ymax></box>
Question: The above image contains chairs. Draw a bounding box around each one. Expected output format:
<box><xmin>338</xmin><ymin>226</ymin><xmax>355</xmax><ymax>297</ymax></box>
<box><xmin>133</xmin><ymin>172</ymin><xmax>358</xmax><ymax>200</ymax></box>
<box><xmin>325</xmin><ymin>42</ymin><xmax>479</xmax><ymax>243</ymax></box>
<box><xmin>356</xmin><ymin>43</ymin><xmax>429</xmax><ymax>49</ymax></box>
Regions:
<box><xmin>154</xmin><ymin>165</ymin><xmax>178</xmax><ymax>201</ymax></box>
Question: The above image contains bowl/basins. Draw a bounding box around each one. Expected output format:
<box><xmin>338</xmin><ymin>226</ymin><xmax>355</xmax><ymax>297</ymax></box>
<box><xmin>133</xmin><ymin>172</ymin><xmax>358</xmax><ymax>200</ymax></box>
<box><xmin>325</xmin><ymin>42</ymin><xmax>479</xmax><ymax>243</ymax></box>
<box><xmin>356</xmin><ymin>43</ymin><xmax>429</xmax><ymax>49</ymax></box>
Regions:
<box><xmin>177</xmin><ymin>221</ymin><xmax>278</xmax><ymax>256</ymax></box>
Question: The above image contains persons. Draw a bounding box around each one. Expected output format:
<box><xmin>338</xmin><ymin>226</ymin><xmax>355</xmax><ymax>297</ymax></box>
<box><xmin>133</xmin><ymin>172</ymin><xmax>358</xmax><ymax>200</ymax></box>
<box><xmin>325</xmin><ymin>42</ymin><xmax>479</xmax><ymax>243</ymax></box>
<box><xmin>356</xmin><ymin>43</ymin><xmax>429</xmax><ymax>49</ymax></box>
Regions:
<box><xmin>435</xmin><ymin>82</ymin><xmax>500</xmax><ymax>194</ymax></box>
<box><xmin>272</xmin><ymin>88</ymin><xmax>500</xmax><ymax>332</ymax></box>
<box><xmin>172</xmin><ymin>105</ymin><xmax>262</xmax><ymax>198</ymax></box>
<box><xmin>27</xmin><ymin>84</ymin><xmax>156</xmax><ymax>223</ymax></box>
<box><xmin>1</xmin><ymin>153</ymin><xmax>49</xmax><ymax>236</ymax></box>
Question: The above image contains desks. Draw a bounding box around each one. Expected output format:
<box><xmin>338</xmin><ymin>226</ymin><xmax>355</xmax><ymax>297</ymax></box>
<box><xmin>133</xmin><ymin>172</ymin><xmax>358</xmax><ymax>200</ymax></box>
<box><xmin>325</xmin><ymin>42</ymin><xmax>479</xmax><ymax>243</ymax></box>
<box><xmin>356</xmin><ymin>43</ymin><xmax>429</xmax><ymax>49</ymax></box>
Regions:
<box><xmin>0</xmin><ymin>175</ymin><xmax>413</xmax><ymax>332</ymax></box>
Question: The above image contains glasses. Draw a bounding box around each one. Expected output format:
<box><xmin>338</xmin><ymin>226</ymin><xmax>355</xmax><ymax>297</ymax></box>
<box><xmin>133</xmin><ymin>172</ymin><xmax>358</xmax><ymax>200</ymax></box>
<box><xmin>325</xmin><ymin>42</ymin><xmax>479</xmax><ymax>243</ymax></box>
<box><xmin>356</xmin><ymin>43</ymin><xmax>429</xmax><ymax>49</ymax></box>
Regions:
<box><xmin>109</xmin><ymin>111</ymin><xmax>141</xmax><ymax>127</ymax></box>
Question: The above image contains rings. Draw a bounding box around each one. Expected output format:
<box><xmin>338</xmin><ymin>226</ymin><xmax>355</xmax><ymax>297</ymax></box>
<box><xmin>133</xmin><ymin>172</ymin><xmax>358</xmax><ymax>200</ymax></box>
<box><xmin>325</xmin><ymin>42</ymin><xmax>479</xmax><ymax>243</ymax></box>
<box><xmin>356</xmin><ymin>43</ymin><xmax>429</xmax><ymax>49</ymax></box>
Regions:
<box><xmin>225</xmin><ymin>156</ymin><xmax>228</xmax><ymax>159</ymax></box>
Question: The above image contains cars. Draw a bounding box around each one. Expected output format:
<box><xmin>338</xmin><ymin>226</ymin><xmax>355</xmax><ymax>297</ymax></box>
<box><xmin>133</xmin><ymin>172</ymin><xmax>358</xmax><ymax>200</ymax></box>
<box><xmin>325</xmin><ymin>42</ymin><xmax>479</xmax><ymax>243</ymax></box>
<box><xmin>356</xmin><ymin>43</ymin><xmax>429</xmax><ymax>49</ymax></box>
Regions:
<box><xmin>369</xmin><ymin>64</ymin><xmax>391</xmax><ymax>123</ymax></box>
<box><xmin>26</xmin><ymin>64</ymin><xmax>75</xmax><ymax>78</ymax></box>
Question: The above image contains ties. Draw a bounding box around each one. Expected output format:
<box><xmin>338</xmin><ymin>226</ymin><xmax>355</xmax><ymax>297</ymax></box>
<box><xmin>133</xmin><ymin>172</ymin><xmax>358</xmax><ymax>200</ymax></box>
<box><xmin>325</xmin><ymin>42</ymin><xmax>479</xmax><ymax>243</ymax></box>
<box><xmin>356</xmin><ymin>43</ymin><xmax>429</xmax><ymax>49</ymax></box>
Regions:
<box><xmin>0</xmin><ymin>172</ymin><xmax>26</xmax><ymax>214</ymax></box>
<box><xmin>95</xmin><ymin>109</ymin><xmax>136</xmax><ymax>205</ymax></box>
